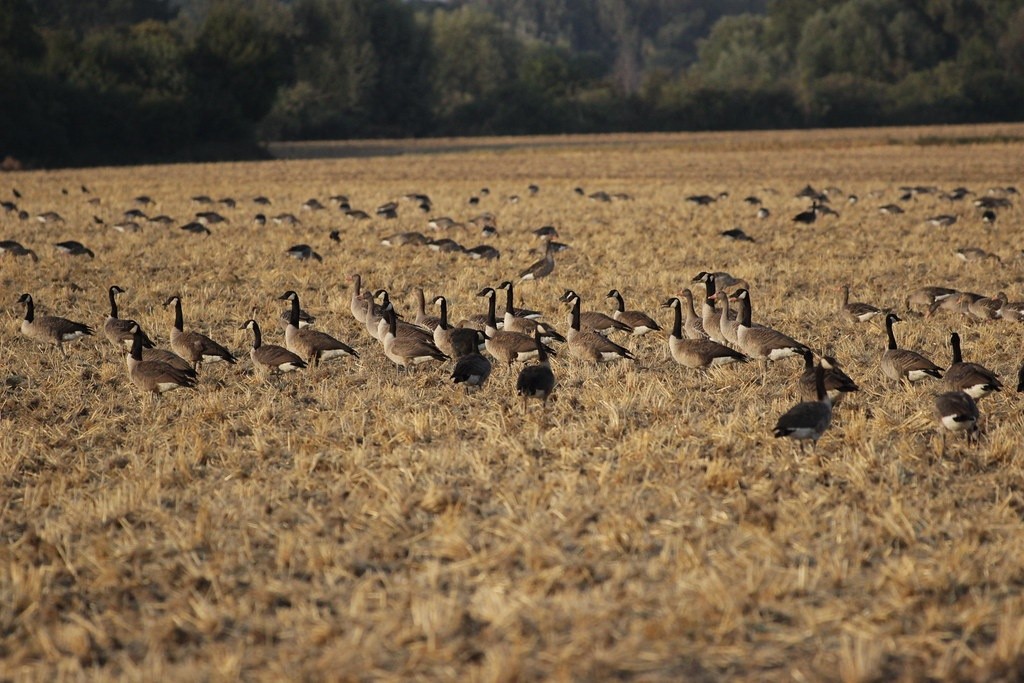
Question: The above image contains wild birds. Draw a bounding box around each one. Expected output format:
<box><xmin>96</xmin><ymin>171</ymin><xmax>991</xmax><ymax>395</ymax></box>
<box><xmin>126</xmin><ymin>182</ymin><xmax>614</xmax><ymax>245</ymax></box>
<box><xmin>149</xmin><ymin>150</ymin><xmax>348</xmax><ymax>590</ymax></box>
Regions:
<box><xmin>0</xmin><ymin>183</ymin><xmax>1024</xmax><ymax>456</ymax></box>
